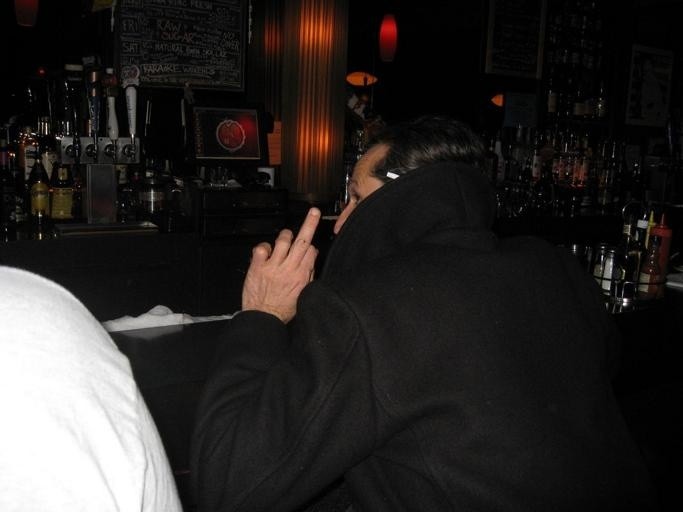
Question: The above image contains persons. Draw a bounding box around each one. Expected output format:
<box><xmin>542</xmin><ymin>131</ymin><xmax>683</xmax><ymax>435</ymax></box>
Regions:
<box><xmin>190</xmin><ymin>107</ymin><xmax>647</xmax><ymax>512</ymax></box>
<box><xmin>1</xmin><ymin>263</ymin><xmax>187</xmax><ymax>511</ymax></box>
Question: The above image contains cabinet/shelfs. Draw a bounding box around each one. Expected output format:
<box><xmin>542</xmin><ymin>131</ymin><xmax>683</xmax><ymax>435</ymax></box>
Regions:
<box><xmin>0</xmin><ymin>186</ymin><xmax>288</xmax><ymax>323</ymax></box>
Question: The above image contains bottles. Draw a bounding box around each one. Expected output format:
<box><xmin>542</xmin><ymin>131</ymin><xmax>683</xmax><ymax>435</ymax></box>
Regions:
<box><xmin>548</xmin><ymin>9</ymin><xmax>616</xmax><ymax>120</ymax></box>
<box><xmin>0</xmin><ymin>66</ymin><xmax>198</xmax><ymax>242</ymax></box>
<box><xmin>329</xmin><ymin>122</ymin><xmax>672</xmax><ymax>314</ymax></box>
